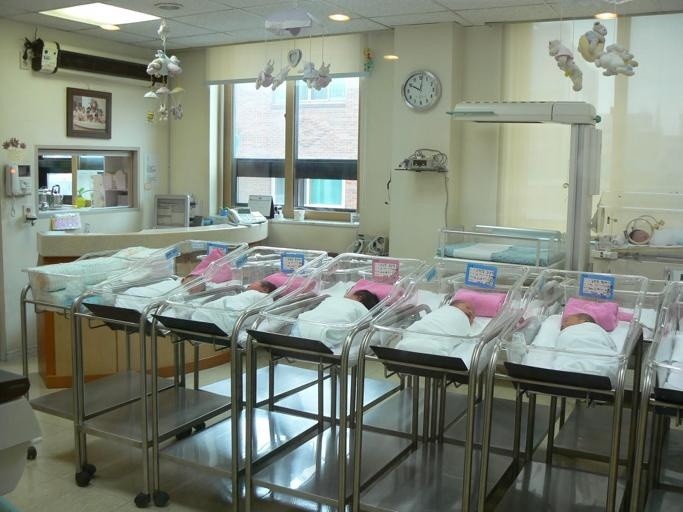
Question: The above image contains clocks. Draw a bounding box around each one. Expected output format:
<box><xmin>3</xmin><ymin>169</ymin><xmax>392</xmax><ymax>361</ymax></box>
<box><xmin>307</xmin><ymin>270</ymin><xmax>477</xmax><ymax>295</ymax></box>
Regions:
<box><xmin>402</xmin><ymin>69</ymin><xmax>442</xmax><ymax>112</ymax></box>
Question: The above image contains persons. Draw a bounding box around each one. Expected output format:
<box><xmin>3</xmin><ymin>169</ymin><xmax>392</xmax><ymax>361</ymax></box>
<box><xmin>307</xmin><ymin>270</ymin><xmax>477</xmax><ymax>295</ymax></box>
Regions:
<box><xmin>192</xmin><ymin>281</ymin><xmax>278</xmax><ymax>333</ymax></box>
<box><xmin>555</xmin><ymin>313</ymin><xmax>619</xmax><ymax>389</ymax></box>
<box><xmin>629</xmin><ymin>226</ymin><xmax>683</xmax><ymax>246</ymax></box>
<box><xmin>74</xmin><ymin>100</ymin><xmax>105</xmax><ymax>123</ymax></box>
<box><xmin>119</xmin><ymin>275</ymin><xmax>205</xmax><ymax>317</ymax></box>
<box><xmin>397</xmin><ymin>300</ymin><xmax>474</xmax><ymax>355</ymax></box>
<box><xmin>291</xmin><ymin>290</ymin><xmax>381</xmax><ymax>349</ymax></box>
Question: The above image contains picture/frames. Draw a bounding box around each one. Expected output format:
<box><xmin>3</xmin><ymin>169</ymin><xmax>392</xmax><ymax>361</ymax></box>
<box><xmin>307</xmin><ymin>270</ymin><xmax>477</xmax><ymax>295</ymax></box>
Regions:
<box><xmin>65</xmin><ymin>87</ymin><xmax>112</xmax><ymax>139</ymax></box>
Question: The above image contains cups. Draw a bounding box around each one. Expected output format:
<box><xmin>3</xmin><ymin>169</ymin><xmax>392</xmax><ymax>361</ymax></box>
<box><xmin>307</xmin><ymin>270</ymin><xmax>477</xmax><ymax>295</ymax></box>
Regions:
<box><xmin>350</xmin><ymin>212</ymin><xmax>359</xmax><ymax>225</ymax></box>
<box><xmin>294</xmin><ymin>210</ymin><xmax>304</xmax><ymax>221</ymax></box>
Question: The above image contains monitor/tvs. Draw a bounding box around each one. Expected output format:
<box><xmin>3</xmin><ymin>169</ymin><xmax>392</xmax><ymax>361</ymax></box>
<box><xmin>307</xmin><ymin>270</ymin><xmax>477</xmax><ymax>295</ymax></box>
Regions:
<box><xmin>155</xmin><ymin>195</ymin><xmax>189</xmax><ymax>229</ymax></box>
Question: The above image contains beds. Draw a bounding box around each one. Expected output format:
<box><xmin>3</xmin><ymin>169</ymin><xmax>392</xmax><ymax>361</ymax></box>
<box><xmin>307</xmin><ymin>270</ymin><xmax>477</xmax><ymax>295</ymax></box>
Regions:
<box><xmin>364</xmin><ymin>256</ymin><xmax>536</xmax><ymax>375</ymax></box>
<box><xmin>248</xmin><ymin>250</ymin><xmax>428</xmax><ymax>359</ymax></box>
<box><xmin>15</xmin><ymin>221</ymin><xmax>329</xmax><ymax>347</ymax></box>
<box><xmin>641</xmin><ymin>279</ymin><xmax>681</xmax><ymax>398</ymax></box>
<box><xmin>494</xmin><ymin>272</ymin><xmax>655</xmax><ymax>389</ymax></box>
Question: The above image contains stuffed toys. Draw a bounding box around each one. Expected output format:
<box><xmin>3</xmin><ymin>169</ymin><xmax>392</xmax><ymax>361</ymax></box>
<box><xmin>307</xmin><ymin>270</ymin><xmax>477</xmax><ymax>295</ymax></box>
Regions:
<box><xmin>548</xmin><ymin>21</ymin><xmax>639</xmax><ymax>91</ymax></box>
<box><xmin>147</xmin><ymin>56</ymin><xmax>182</xmax><ymax>78</ymax></box>
<box><xmin>256</xmin><ymin>59</ymin><xmax>291</xmax><ymax>90</ymax></box>
<box><xmin>147</xmin><ymin>105</ymin><xmax>184</xmax><ymax>123</ymax></box>
<box><xmin>297</xmin><ymin>61</ymin><xmax>331</xmax><ymax>90</ymax></box>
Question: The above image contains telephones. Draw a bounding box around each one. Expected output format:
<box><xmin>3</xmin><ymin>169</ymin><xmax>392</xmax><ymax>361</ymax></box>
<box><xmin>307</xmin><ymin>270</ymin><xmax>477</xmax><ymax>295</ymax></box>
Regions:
<box><xmin>229</xmin><ymin>207</ymin><xmax>267</xmax><ymax>225</ymax></box>
<box><xmin>6</xmin><ymin>164</ymin><xmax>33</xmax><ymax>197</ymax></box>
